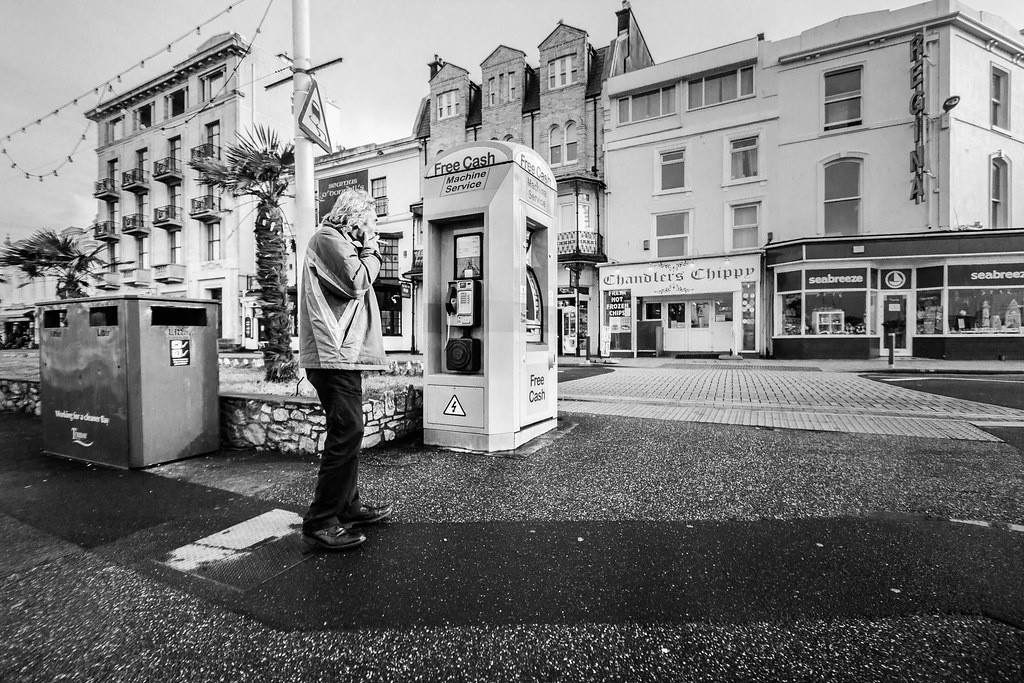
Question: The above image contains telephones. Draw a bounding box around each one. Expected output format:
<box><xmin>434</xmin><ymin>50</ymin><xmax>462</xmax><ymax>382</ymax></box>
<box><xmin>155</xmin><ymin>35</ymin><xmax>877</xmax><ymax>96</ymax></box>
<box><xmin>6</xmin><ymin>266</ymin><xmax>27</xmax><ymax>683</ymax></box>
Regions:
<box><xmin>446</xmin><ymin>280</ymin><xmax>481</xmax><ymax>327</ymax></box>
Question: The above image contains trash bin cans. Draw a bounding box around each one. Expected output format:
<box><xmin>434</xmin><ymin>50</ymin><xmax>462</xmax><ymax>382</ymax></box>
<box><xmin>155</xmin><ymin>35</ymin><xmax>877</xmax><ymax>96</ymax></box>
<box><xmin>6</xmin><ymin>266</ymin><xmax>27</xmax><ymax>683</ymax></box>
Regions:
<box><xmin>33</xmin><ymin>294</ymin><xmax>222</xmax><ymax>470</ymax></box>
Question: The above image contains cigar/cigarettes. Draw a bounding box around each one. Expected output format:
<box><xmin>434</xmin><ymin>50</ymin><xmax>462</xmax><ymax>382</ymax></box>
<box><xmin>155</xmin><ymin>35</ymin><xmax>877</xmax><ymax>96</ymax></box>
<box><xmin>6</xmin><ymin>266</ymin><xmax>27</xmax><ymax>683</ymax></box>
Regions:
<box><xmin>377</xmin><ymin>240</ymin><xmax>389</xmax><ymax>245</ymax></box>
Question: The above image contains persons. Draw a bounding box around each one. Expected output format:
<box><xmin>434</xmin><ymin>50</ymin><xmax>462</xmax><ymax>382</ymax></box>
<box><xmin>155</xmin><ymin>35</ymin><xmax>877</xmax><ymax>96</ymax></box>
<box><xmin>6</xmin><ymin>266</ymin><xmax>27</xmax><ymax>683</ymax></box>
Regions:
<box><xmin>298</xmin><ymin>186</ymin><xmax>394</xmax><ymax>551</ymax></box>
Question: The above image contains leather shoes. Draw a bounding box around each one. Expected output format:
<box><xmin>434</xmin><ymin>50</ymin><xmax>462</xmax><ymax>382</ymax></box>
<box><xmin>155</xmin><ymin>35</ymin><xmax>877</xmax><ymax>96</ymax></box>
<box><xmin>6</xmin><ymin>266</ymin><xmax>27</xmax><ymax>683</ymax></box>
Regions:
<box><xmin>338</xmin><ymin>505</ymin><xmax>393</xmax><ymax>524</ymax></box>
<box><xmin>301</xmin><ymin>523</ymin><xmax>366</xmax><ymax>547</ymax></box>
